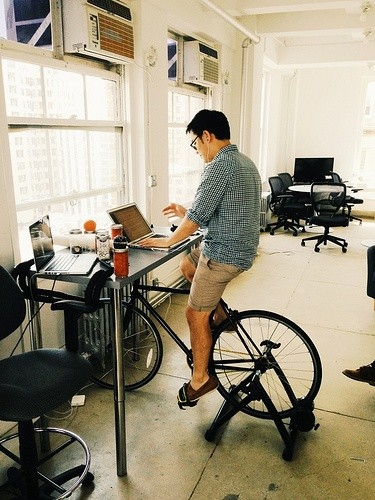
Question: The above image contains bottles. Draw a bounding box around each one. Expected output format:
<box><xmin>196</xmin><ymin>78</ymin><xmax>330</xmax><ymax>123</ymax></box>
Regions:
<box><xmin>69</xmin><ymin>228</ymin><xmax>111</xmax><ymax>261</ymax></box>
<box><xmin>113</xmin><ymin>236</ymin><xmax>129</xmax><ymax>279</ymax></box>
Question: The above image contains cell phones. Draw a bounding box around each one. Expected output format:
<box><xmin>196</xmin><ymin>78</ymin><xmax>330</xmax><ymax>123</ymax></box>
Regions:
<box><xmin>100</xmin><ymin>259</ymin><xmax>114</xmax><ymax>267</ymax></box>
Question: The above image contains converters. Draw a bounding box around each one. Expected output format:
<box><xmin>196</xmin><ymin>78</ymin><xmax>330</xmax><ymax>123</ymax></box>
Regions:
<box><xmin>69</xmin><ymin>394</ymin><xmax>86</xmax><ymax>407</ymax></box>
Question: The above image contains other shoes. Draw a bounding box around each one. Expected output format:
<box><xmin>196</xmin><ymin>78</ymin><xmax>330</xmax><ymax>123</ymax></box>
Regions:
<box><xmin>177</xmin><ymin>374</ymin><xmax>219</xmax><ymax>403</ymax></box>
<box><xmin>210</xmin><ymin>323</ymin><xmax>236</xmax><ymax>333</ymax></box>
<box><xmin>342</xmin><ymin>361</ymin><xmax>375</xmax><ymax>386</ymax></box>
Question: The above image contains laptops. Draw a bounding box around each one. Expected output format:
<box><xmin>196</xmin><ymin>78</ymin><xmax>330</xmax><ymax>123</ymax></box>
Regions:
<box><xmin>29</xmin><ymin>214</ymin><xmax>99</xmax><ymax>276</ymax></box>
<box><xmin>107</xmin><ymin>202</ymin><xmax>190</xmax><ymax>251</ymax></box>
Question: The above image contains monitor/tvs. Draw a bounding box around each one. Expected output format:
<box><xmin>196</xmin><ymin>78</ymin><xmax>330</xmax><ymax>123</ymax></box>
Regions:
<box><xmin>293</xmin><ymin>158</ymin><xmax>334</xmax><ymax>184</ymax></box>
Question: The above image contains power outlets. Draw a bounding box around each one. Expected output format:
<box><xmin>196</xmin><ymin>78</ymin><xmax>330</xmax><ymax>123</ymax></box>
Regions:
<box><xmin>152</xmin><ymin>278</ymin><xmax>159</xmax><ymax>287</ymax></box>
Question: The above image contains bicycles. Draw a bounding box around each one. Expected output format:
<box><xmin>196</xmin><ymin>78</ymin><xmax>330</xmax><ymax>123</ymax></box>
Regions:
<box><xmin>63</xmin><ymin>276</ymin><xmax>322</xmax><ymax>422</ymax></box>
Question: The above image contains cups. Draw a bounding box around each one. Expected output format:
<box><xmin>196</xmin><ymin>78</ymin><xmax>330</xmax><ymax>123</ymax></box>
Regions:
<box><xmin>110</xmin><ymin>223</ymin><xmax>123</xmax><ymax>239</ymax></box>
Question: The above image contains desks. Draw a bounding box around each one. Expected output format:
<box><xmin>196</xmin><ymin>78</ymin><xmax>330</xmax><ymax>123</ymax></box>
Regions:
<box><xmin>28</xmin><ymin>229</ymin><xmax>203</xmax><ymax>477</ymax></box>
<box><xmin>288</xmin><ymin>183</ymin><xmax>344</xmax><ymax>200</ymax></box>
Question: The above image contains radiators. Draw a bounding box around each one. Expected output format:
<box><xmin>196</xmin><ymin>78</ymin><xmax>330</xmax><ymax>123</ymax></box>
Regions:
<box><xmin>259</xmin><ymin>192</ymin><xmax>271</xmax><ymax>231</ymax></box>
<box><xmin>80</xmin><ymin>275</ymin><xmax>147</xmax><ymax>352</ymax></box>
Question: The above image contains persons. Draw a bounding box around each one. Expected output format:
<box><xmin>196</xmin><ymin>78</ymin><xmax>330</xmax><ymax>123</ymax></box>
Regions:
<box><xmin>137</xmin><ymin>109</ymin><xmax>262</xmax><ymax>401</ymax></box>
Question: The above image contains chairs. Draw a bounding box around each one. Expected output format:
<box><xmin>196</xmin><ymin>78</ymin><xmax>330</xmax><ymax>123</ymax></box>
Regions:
<box><xmin>0</xmin><ymin>265</ymin><xmax>94</xmax><ymax>500</ymax></box>
<box><xmin>265</xmin><ymin>171</ymin><xmax>363</xmax><ymax>251</ymax></box>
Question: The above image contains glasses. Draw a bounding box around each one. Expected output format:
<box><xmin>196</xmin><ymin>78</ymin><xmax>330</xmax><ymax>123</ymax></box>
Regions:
<box><xmin>190</xmin><ymin>131</ymin><xmax>202</xmax><ymax>150</ymax></box>
